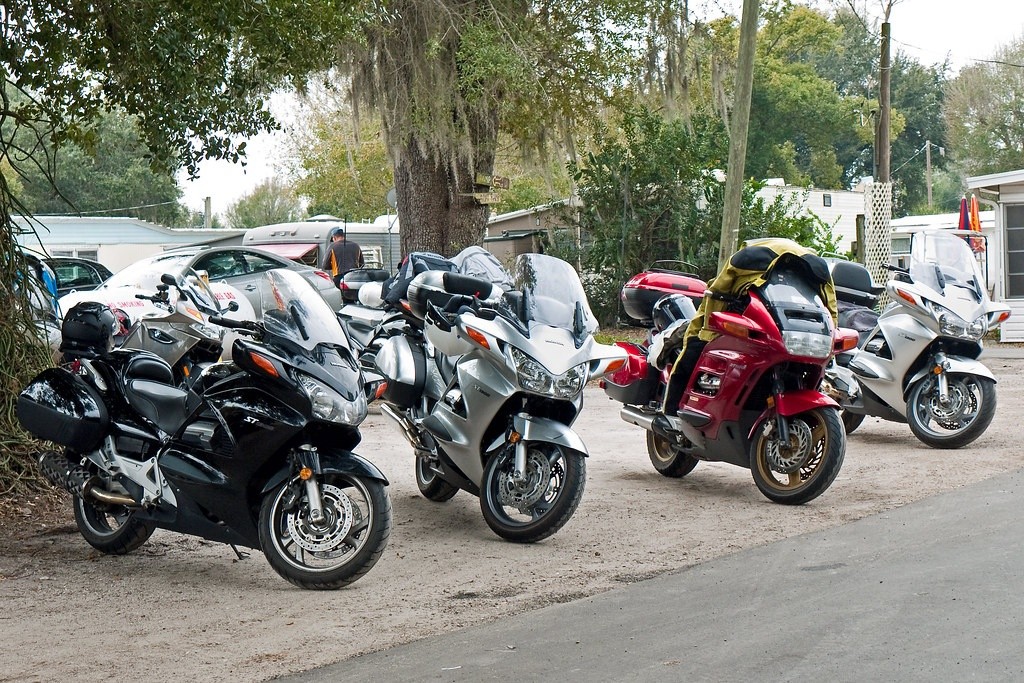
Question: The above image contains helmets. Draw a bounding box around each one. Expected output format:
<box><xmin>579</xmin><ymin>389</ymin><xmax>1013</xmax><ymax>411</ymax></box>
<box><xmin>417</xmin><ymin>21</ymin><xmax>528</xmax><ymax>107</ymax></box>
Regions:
<box><xmin>652</xmin><ymin>294</ymin><xmax>698</xmax><ymax>333</ymax></box>
<box><xmin>358</xmin><ymin>281</ymin><xmax>386</xmax><ymax>310</ymax></box>
<box><xmin>59</xmin><ymin>302</ymin><xmax>121</xmax><ymax>357</ymax></box>
<box><xmin>425</xmin><ymin>298</ymin><xmax>475</xmax><ymax>356</ymax></box>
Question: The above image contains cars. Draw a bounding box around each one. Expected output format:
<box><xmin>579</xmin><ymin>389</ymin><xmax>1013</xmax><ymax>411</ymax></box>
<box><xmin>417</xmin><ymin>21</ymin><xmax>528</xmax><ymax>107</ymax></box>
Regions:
<box><xmin>12</xmin><ymin>257</ymin><xmax>114</xmax><ymax>317</ymax></box>
<box><xmin>57</xmin><ymin>246</ymin><xmax>341</xmax><ymax>330</ymax></box>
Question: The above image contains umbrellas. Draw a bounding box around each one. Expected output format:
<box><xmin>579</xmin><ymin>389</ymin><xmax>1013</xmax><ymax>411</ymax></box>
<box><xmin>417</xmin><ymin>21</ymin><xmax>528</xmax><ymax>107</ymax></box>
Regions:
<box><xmin>958</xmin><ymin>193</ymin><xmax>985</xmax><ymax>258</ymax></box>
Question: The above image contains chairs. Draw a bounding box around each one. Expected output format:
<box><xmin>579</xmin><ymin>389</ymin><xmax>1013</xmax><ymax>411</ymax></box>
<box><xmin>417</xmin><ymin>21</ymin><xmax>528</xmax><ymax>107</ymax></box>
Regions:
<box><xmin>207</xmin><ymin>264</ymin><xmax>225</xmax><ymax>276</ymax></box>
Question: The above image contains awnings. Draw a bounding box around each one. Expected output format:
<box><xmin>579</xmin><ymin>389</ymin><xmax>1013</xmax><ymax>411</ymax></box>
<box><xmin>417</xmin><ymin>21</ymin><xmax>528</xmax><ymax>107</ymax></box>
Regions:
<box><xmin>485</xmin><ymin>230</ymin><xmax>536</xmax><ymax>243</ymax></box>
<box><xmin>254</xmin><ymin>244</ymin><xmax>319</xmax><ymax>259</ymax></box>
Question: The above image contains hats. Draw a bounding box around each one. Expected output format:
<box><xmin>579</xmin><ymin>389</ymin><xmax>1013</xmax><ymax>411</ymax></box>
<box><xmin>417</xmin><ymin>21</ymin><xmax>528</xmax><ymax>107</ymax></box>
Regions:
<box><xmin>330</xmin><ymin>229</ymin><xmax>344</xmax><ymax>242</ymax></box>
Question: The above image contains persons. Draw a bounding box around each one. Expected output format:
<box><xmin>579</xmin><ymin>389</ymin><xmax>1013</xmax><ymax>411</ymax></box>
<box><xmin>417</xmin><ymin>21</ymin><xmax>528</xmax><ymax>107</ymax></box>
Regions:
<box><xmin>322</xmin><ymin>228</ymin><xmax>364</xmax><ymax>289</ymax></box>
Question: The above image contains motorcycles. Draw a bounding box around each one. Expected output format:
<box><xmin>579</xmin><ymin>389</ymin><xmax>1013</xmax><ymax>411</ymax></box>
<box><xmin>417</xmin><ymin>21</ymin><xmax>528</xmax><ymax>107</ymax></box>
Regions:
<box><xmin>16</xmin><ymin>269</ymin><xmax>391</xmax><ymax>590</ymax></box>
<box><xmin>22</xmin><ymin>266</ymin><xmax>238</xmax><ymax>374</ymax></box>
<box><xmin>819</xmin><ymin>230</ymin><xmax>1012</xmax><ymax>449</ymax></box>
<box><xmin>599</xmin><ymin>238</ymin><xmax>847</xmax><ymax>505</ymax></box>
<box><xmin>335</xmin><ymin>246</ymin><xmax>628</xmax><ymax>541</ymax></box>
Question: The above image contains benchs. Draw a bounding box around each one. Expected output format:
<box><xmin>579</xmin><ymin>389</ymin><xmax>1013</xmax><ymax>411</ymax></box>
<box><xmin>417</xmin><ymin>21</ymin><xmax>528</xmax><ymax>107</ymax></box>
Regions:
<box><xmin>68</xmin><ymin>277</ymin><xmax>89</xmax><ymax>285</ymax></box>
